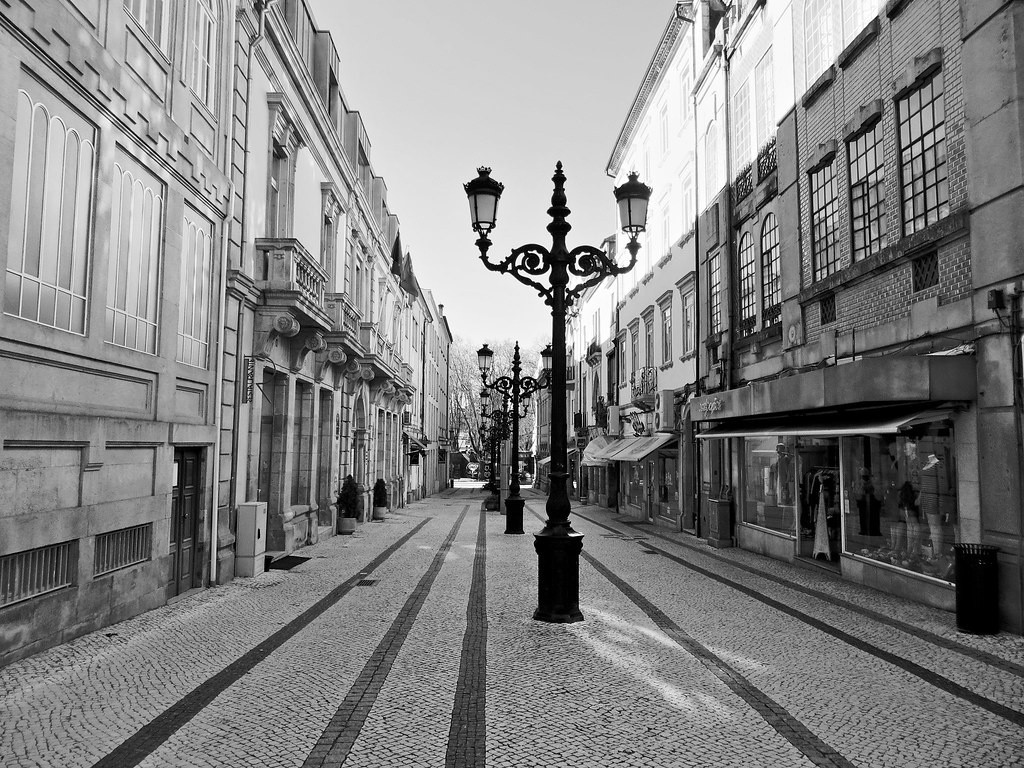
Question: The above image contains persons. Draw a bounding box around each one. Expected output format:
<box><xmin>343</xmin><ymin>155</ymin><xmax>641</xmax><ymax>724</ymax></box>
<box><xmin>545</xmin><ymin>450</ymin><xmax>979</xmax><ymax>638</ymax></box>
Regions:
<box><xmin>885</xmin><ymin>442</ymin><xmax>950</xmax><ymax>567</ymax></box>
<box><xmin>774</xmin><ymin>443</ymin><xmax>795</xmax><ymax>531</ymax></box>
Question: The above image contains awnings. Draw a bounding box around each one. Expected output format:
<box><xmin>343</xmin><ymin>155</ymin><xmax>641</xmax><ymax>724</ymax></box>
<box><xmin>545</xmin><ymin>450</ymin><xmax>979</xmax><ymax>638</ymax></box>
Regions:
<box><xmin>581</xmin><ymin>434</ymin><xmax>676</xmax><ymax>467</ymax></box>
<box><xmin>402</xmin><ymin>431</ymin><xmax>429</xmax><ymax>457</ymax></box>
<box><xmin>695</xmin><ymin>410</ymin><xmax>953</xmax><ymax>438</ymax></box>
<box><xmin>537</xmin><ymin>448</ymin><xmax>576</xmax><ymax>467</ymax></box>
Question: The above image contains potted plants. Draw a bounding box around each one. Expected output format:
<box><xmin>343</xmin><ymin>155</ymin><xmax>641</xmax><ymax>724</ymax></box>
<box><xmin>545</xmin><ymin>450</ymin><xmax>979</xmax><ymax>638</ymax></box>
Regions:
<box><xmin>373</xmin><ymin>479</ymin><xmax>387</xmax><ymax>520</ymax></box>
<box><xmin>336</xmin><ymin>474</ymin><xmax>361</xmax><ymax>535</ymax></box>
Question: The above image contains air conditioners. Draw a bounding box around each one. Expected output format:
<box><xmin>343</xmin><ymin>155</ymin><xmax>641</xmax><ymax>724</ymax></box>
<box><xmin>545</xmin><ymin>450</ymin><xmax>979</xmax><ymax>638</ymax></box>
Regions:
<box><xmin>607</xmin><ymin>406</ymin><xmax>619</xmax><ymax>435</ymax></box>
<box><xmin>654</xmin><ymin>390</ymin><xmax>675</xmax><ymax>431</ymax></box>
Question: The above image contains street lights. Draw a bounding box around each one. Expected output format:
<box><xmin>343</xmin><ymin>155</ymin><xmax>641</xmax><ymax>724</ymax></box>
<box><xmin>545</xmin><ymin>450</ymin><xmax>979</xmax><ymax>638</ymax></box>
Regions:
<box><xmin>479</xmin><ymin>388</ymin><xmax>532</xmax><ymax>439</ymax></box>
<box><xmin>480</xmin><ymin>421</ymin><xmax>513</xmax><ymax>464</ymax></box>
<box><xmin>462</xmin><ymin>160</ymin><xmax>653</xmax><ymax>624</ymax></box>
<box><xmin>476</xmin><ymin>341</ymin><xmax>553</xmax><ymax>534</ymax></box>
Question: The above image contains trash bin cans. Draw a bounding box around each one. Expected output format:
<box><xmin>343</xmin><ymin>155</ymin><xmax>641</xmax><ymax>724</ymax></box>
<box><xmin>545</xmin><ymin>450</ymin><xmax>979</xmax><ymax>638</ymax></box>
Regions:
<box><xmin>951</xmin><ymin>542</ymin><xmax>1003</xmax><ymax>636</ymax></box>
